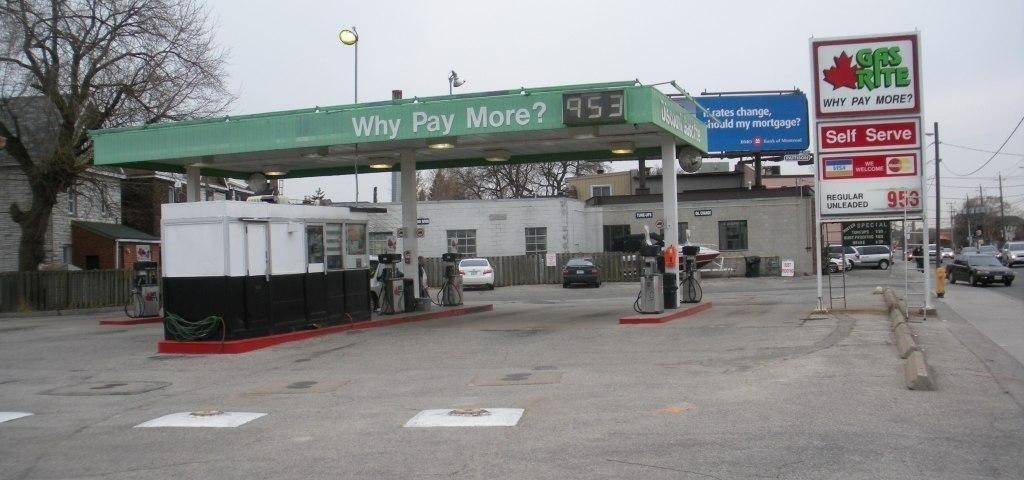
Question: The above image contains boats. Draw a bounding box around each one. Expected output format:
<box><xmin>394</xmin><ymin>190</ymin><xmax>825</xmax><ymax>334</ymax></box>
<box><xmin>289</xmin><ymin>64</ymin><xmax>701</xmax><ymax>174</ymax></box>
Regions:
<box><xmin>613</xmin><ymin>232</ymin><xmax>720</xmax><ymax>273</ymax></box>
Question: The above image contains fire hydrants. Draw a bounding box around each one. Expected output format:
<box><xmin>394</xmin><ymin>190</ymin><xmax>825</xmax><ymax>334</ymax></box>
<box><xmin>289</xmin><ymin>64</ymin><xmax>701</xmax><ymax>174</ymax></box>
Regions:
<box><xmin>935</xmin><ymin>267</ymin><xmax>946</xmax><ymax>298</ymax></box>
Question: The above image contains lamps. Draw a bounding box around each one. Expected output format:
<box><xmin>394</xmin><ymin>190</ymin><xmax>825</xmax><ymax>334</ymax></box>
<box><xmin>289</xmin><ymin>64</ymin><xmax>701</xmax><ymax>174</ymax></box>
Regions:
<box><xmin>609</xmin><ymin>141</ymin><xmax>635</xmax><ymax>154</ymax></box>
<box><xmin>188</xmin><ymin>157</ymin><xmax>213</xmax><ymax>168</ymax></box>
<box><xmin>263</xmin><ymin>164</ymin><xmax>288</xmax><ymax>176</ymax></box>
<box><xmin>340</xmin><ymin>25</ymin><xmax>360</xmax><ymax>107</ymax></box>
<box><xmin>369</xmin><ymin>157</ymin><xmax>396</xmax><ymax>168</ymax></box>
<box><xmin>302</xmin><ymin>147</ymin><xmax>326</xmax><ymax>159</ymax></box>
<box><xmin>427</xmin><ymin>137</ymin><xmax>455</xmax><ymax>150</ymax></box>
<box><xmin>484</xmin><ymin>149</ymin><xmax>510</xmax><ymax>162</ymax></box>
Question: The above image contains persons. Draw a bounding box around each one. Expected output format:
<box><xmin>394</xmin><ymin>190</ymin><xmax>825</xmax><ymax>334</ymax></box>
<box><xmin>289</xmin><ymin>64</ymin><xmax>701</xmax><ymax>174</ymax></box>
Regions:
<box><xmin>909</xmin><ymin>243</ymin><xmax>924</xmax><ymax>273</ymax></box>
<box><xmin>418</xmin><ymin>255</ymin><xmax>427</xmax><ymax>299</ymax></box>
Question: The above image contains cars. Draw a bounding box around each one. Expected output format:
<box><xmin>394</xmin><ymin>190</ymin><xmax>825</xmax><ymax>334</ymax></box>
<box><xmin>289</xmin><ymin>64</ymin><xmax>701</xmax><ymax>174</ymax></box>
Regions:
<box><xmin>824</xmin><ymin>243</ymin><xmax>893</xmax><ymax>274</ymax></box>
<box><xmin>922</xmin><ymin>240</ymin><xmax>1024</xmax><ymax>287</ymax></box>
<box><xmin>457</xmin><ymin>258</ymin><xmax>496</xmax><ymax>290</ymax></box>
<box><xmin>560</xmin><ymin>258</ymin><xmax>601</xmax><ymax>289</ymax></box>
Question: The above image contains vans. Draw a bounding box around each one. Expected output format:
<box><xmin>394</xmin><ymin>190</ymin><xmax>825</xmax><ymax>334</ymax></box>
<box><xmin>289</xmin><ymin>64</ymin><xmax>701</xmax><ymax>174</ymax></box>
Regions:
<box><xmin>369</xmin><ymin>254</ymin><xmax>430</xmax><ymax>314</ymax></box>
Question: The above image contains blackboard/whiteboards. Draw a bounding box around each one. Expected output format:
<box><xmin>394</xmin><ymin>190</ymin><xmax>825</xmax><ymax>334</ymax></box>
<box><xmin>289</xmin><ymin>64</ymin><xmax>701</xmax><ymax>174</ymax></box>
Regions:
<box><xmin>841</xmin><ymin>221</ymin><xmax>891</xmax><ymax>247</ymax></box>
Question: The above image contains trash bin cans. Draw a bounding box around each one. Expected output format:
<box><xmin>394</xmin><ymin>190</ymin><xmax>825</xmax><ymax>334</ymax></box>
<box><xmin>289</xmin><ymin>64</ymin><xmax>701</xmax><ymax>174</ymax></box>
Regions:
<box><xmin>745</xmin><ymin>256</ymin><xmax>760</xmax><ymax>277</ymax></box>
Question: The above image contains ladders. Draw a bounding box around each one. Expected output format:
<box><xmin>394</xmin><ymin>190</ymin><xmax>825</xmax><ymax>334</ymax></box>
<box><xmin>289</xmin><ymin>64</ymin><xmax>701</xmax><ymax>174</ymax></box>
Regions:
<box><xmin>826</xmin><ymin>222</ymin><xmax>846</xmax><ymax>310</ymax></box>
<box><xmin>903</xmin><ymin>188</ymin><xmax>928</xmax><ymax>320</ymax></box>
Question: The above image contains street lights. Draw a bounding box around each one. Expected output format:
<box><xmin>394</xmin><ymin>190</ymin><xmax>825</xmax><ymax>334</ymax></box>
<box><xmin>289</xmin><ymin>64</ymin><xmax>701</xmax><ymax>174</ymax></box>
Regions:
<box><xmin>337</xmin><ymin>25</ymin><xmax>359</xmax><ymax>105</ymax></box>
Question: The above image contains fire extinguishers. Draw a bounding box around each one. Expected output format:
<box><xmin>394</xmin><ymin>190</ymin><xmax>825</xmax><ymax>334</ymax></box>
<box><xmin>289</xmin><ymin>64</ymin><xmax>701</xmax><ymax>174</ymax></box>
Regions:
<box><xmin>403</xmin><ymin>250</ymin><xmax>411</xmax><ymax>265</ymax></box>
